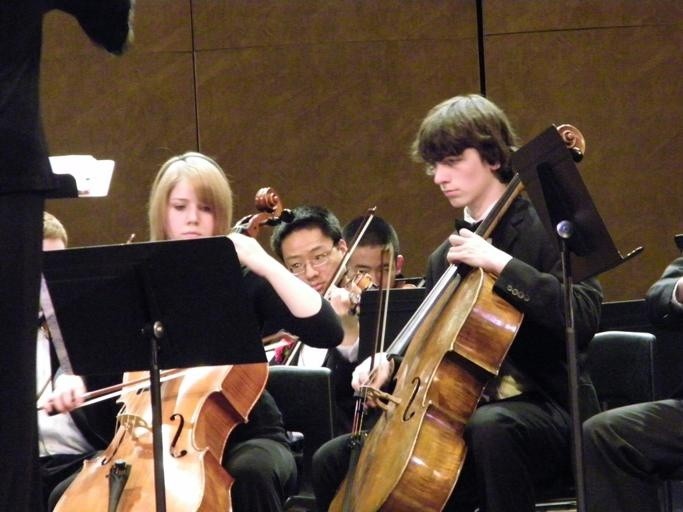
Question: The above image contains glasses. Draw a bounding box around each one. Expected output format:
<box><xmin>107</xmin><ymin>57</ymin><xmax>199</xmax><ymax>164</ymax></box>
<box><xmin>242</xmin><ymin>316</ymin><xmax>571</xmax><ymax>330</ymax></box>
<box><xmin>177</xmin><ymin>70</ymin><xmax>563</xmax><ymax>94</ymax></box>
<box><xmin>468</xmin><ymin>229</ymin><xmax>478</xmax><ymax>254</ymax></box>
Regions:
<box><xmin>285</xmin><ymin>245</ymin><xmax>335</xmax><ymax>275</ymax></box>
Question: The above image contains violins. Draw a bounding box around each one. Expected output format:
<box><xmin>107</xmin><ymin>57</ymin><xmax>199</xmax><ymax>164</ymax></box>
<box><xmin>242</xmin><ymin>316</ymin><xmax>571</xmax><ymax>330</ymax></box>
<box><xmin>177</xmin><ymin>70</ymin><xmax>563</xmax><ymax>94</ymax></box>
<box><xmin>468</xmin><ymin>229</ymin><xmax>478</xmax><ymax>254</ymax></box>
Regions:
<box><xmin>251</xmin><ymin>268</ymin><xmax>376</xmax><ymax>380</ymax></box>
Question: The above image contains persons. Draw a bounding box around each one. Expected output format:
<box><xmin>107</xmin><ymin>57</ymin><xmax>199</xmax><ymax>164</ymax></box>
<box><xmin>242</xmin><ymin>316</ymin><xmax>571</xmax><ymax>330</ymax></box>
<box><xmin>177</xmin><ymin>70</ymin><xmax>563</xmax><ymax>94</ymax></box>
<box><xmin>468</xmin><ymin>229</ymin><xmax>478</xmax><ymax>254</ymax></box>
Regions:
<box><xmin>37</xmin><ymin>207</ymin><xmax>93</xmax><ymax>491</ymax></box>
<box><xmin>268</xmin><ymin>203</ymin><xmax>363</xmax><ymax>425</ymax></box>
<box><xmin>580</xmin><ymin>249</ymin><xmax>683</xmax><ymax>512</ymax></box>
<box><xmin>341</xmin><ymin>211</ymin><xmax>426</xmax><ymax>310</ymax></box>
<box><xmin>43</xmin><ymin>153</ymin><xmax>345</xmax><ymax>512</ymax></box>
<box><xmin>307</xmin><ymin>90</ymin><xmax>604</xmax><ymax>512</ymax></box>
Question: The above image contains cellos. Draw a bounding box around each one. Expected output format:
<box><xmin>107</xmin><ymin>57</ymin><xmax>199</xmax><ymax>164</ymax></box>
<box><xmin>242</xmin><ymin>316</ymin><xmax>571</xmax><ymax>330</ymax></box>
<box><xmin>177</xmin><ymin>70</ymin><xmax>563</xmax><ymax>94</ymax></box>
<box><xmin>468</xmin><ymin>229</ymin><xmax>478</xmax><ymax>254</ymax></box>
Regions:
<box><xmin>50</xmin><ymin>185</ymin><xmax>285</xmax><ymax>507</ymax></box>
<box><xmin>327</xmin><ymin>121</ymin><xmax>588</xmax><ymax>503</ymax></box>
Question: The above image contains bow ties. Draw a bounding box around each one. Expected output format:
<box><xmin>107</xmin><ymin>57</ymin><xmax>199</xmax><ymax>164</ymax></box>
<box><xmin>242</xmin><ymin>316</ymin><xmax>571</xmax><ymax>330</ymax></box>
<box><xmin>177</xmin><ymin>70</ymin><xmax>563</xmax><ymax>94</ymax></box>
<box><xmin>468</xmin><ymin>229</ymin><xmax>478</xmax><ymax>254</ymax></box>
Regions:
<box><xmin>453</xmin><ymin>219</ymin><xmax>483</xmax><ymax>236</ymax></box>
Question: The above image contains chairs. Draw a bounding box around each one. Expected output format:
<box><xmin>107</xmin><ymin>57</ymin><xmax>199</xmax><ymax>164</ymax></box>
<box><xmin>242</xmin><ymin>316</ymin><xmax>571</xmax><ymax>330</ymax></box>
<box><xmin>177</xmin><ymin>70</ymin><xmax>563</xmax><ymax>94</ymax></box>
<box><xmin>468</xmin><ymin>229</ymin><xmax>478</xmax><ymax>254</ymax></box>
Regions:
<box><xmin>262</xmin><ymin>365</ymin><xmax>332</xmax><ymax>512</ymax></box>
<box><xmin>527</xmin><ymin>331</ymin><xmax>657</xmax><ymax>507</ymax></box>
